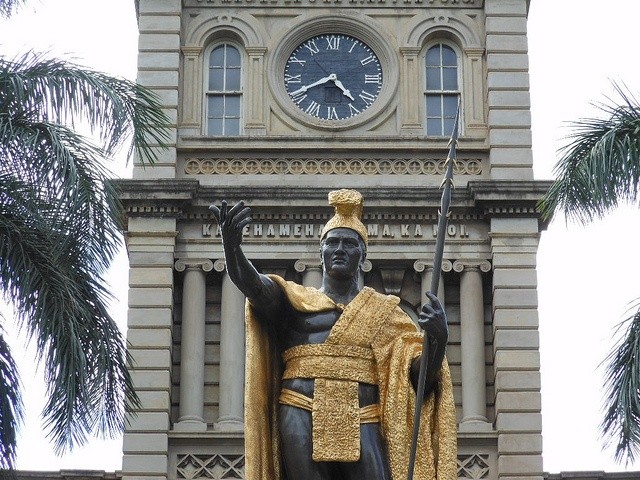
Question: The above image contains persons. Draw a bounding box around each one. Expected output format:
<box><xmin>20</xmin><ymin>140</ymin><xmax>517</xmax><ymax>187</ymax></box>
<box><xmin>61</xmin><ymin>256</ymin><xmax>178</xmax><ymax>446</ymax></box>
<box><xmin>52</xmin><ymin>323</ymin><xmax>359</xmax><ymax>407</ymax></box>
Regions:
<box><xmin>208</xmin><ymin>187</ymin><xmax>458</xmax><ymax>480</ymax></box>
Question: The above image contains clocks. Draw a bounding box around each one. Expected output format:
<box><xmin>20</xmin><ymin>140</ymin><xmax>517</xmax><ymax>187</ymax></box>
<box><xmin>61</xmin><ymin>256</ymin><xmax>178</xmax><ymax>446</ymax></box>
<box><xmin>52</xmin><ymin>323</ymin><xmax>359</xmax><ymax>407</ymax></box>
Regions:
<box><xmin>283</xmin><ymin>33</ymin><xmax>383</xmax><ymax>121</ymax></box>
